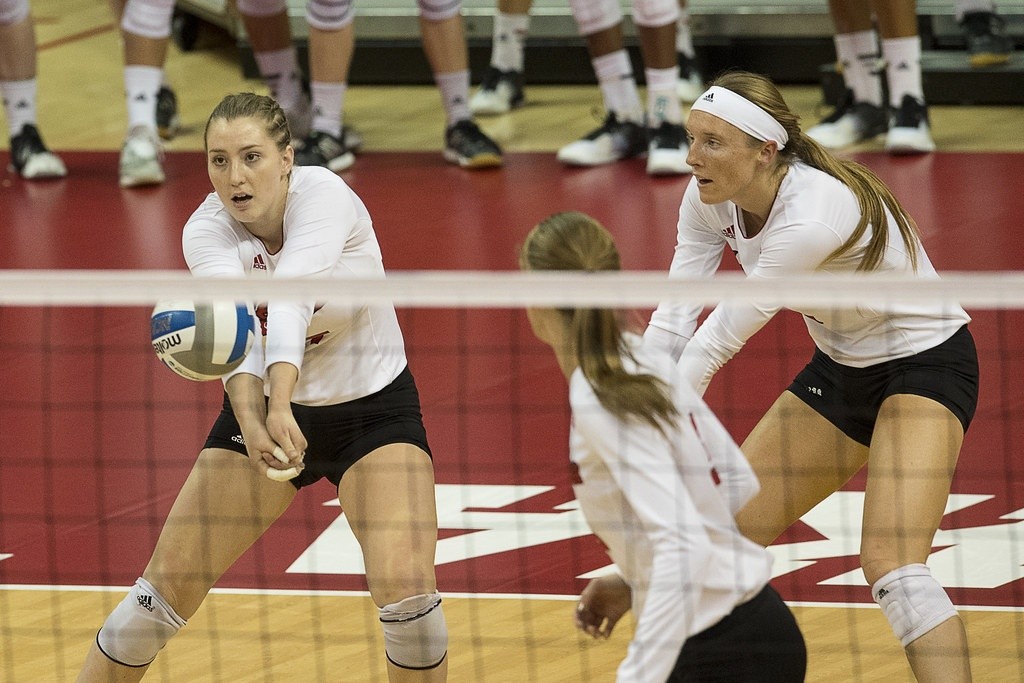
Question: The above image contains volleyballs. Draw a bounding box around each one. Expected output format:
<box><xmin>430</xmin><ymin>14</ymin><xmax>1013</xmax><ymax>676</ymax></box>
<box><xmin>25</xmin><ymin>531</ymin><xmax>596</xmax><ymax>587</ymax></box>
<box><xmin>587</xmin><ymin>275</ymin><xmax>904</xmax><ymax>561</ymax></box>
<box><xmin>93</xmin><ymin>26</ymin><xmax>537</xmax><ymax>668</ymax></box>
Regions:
<box><xmin>148</xmin><ymin>300</ymin><xmax>257</xmax><ymax>382</ymax></box>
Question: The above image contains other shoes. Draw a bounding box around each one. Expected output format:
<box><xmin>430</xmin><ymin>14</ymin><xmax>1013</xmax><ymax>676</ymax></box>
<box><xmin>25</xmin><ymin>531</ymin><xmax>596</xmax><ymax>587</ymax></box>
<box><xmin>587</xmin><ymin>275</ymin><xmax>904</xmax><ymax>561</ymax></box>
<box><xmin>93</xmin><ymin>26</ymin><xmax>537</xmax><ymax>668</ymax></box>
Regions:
<box><xmin>470</xmin><ymin>66</ymin><xmax>524</xmax><ymax>115</ymax></box>
<box><xmin>157</xmin><ymin>87</ymin><xmax>182</xmax><ymax>139</ymax></box>
<box><xmin>119</xmin><ymin>125</ymin><xmax>164</xmax><ymax>186</ymax></box>
<box><xmin>960</xmin><ymin>11</ymin><xmax>1009</xmax><ymax>64</ymax></box>
<box><xmin>885</xmin><ymin>94</ymin><xmax>935</xmax><ymax>152</ymax></box>
<box><xmin>808</xmin><ymin>89</ymin><xmax>887</xmax><ymax>147</ymax></box>
<box><xmin>12</xmin><ymin>125</ymin><xmax>66</xmax><ymax>177</ymax></box>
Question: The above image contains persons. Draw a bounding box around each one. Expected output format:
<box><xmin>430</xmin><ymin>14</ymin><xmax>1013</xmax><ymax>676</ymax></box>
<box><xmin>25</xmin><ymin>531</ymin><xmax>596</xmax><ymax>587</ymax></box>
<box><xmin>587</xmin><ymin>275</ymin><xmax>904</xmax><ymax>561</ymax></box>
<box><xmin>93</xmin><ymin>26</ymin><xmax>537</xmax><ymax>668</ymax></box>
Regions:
<box><xmin>516</xmin><ymin>211</ymin><xmax>808</xmax><ymax>683</ymax></box>
<box><xmin>74</xmin><ymin>93</ymin><xmax>448</xmax><ymax>683</ymax></box>
<box><xmin>803</xmin><ymin>0</ymin><xmax>937</xmax><ymax>155</ymax></box>
<box><xmin>0</xmin><ymin>0</ymin><xmax>706</xmax><ymax>174</ymax></box>
<box><xmin>644</xmin><ymin>70</ymin><xmax>978</xmax><ymax>683</ymax></box>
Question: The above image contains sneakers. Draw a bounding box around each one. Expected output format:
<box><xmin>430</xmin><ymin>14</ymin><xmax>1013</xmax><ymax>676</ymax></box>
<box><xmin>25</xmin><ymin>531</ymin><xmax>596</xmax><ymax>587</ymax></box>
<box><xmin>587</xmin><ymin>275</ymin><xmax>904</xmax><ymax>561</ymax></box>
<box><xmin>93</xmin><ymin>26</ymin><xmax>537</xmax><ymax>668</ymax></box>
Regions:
<box><xmin>647</xmin><ymin>121</ymin><xmax>689</xmax><ymax>175</ymax></box>
<box><xmin>443</xmin><ymin>120</ymin><xmax>503</xmax><ymax>168</ymax></box>
<box><xmin>295</xmin><ymin>129</ymin><xmax>355</xmax><ymax>172</ymax></box>
<box><xmin>557</xmin><ymin>106</ymin><xmax>646</xmax><ymax>166</ymax></box>
